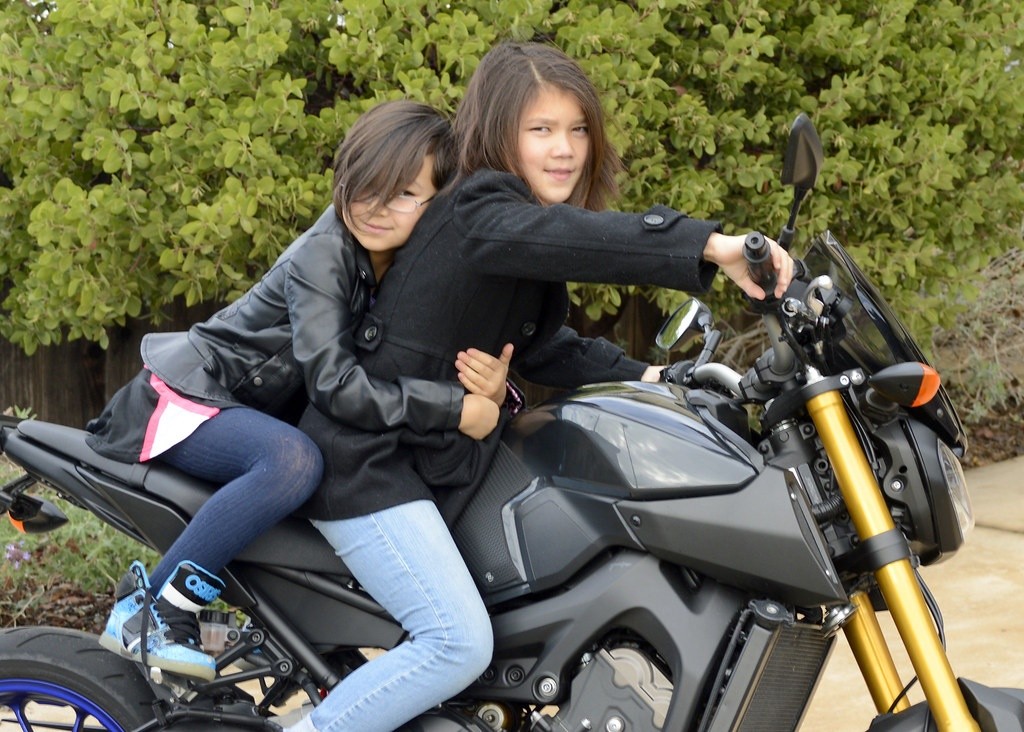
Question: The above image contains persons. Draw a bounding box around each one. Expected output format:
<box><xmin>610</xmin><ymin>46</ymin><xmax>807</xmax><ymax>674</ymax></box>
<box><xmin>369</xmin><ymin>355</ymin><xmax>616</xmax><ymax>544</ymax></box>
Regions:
<box><xmin>87</xmin><ymin>101</ymin><xmax>514</xmax><ymax>683</ymax></box>
<box><xmin>284</xmin><ymin>41</ymin><xmax>795</xmax><ymax>732</ymax></box>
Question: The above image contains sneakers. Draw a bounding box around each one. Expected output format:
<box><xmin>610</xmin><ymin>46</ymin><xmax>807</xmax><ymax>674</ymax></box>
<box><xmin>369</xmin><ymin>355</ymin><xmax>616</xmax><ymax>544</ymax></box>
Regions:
<box><xmin>98</xmin><ymin>560</ymin><xmax>225</xmax><ymax>684</ymax></box>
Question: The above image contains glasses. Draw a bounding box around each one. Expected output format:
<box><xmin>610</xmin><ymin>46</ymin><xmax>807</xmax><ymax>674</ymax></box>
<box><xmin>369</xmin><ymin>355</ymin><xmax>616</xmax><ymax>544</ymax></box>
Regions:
<box><xmin>339</xmin><ymin>184</ymin><xmax>438</xmax><ymax>214</ymax></box>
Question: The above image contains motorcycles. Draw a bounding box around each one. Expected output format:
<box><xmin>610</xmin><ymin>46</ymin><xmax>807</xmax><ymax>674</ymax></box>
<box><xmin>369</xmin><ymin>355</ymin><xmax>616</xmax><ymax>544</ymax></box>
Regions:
<box><xmin>1</xmin><ymin>115</ymin><xmax>1024</xmax><ymax>731</ymax></box>
